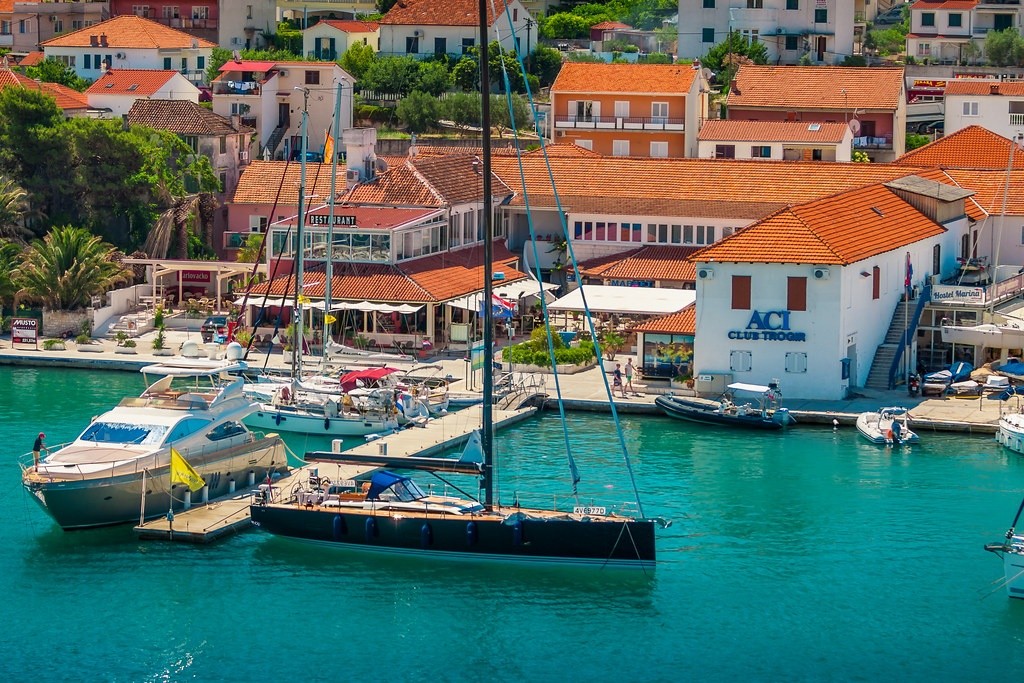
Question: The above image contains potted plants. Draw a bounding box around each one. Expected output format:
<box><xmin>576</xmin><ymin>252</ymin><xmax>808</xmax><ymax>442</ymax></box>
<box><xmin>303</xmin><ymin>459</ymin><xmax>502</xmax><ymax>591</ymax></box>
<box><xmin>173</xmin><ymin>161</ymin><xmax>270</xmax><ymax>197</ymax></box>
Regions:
<box><xmin>76</xmin><ymin>335</ymin><xmax>104</xmax><ymax>352</ymax></box>
<box><xmin>152</xmin><ymin>331</ymin><xmax>172</xmax><ymax>355</ymax></box>
<box><xmin>236</xmin><ymin>327</ymin><xmax>251</xmax><ymax>359</ymax></box>
<box><xmin>42</xmin><ymin>340</ymin><xmax>66</xmax><ymax>350</ymax></box>
<box><xmin>661</xmin><ymin>343</ymin><xmax>693</xmax><ymax>389</ymax></box>
<box><xmin>283</xmin><ymin>345</ymin><xmax>298</xmax><ymax>363</ymax></box>
<box><xmin>599</xmin><ymin>332</ymin><xmax>624</xmax><ymax>373</ymax></box>
<box><xmin>114</xmin><ymin>330</ymin><xmax>137</xmax><ymax>353</ymax></box>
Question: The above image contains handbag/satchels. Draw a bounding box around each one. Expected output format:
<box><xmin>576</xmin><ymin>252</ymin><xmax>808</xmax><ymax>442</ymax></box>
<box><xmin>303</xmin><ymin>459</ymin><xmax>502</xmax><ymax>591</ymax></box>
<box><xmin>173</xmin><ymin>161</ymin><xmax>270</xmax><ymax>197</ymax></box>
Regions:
<box><xmin>614</xmin><ymin>382</ymin><xmax>619</xmax><ymax>386</ymax></box>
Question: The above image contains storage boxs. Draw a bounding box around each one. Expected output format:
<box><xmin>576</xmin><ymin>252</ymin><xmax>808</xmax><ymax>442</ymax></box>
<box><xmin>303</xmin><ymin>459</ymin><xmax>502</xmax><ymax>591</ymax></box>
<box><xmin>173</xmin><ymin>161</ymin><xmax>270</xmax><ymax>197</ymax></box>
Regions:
<box><xmin>696</xmin><ymin>374</ymin><xmax>733</xmax><ymax>400</ymax></box>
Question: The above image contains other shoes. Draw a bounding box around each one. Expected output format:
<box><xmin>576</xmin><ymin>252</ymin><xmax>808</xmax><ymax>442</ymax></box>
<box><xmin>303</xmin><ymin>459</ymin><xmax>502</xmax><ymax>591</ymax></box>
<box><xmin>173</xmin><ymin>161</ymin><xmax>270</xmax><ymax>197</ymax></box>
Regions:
<box><xmin>622</xmin><ymin>394</ymin><xmax>626</xmax><ymax>396</ymax></box>
<box><xmin>632</xmin><ymin>393</ymin><xmax>637</xmax><ymax>396</ymax></box>
<box><xmin>612</xmin><ymin>394</ymin><xmax>615</xmax><ymax>397</ymax></box>
<box><xmin>624</xmin><ymin>392</ymin><xmax>628</xmax><ymax>394</ymax></box>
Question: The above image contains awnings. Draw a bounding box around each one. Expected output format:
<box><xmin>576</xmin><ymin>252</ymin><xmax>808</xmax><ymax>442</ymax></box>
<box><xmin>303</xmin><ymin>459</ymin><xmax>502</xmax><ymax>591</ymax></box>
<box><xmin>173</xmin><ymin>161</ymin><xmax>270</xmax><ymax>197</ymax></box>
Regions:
<box><xmin>443</xmin><ymin>278</ymin><xmax>562</xmax><ymax>323</ymax></box>
<box><xmin>230</xmin><ymin>294</ymin><xmax>426</xmax><ymax>327</ymax></box>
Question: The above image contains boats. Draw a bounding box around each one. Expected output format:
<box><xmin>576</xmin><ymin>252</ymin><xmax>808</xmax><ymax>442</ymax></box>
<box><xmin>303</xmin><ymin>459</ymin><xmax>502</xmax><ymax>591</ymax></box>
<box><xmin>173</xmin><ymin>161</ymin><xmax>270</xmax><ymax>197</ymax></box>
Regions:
<box><xmin>982</xmin><ymin>486</ymin><xmax>1024</xmax><ymax>600</ymax></box>
<box><xmin>17</xmin><ymin>343</ymin><xmax>291</xmax><ymax>531</ymax></box>
<box><xmin>996</xmin><ymin>412</ymin><xmax>1024</xmax><ymax>455</ymax></box>
<box><xmin>856</xmin><ymin>409</ymin><xmax>918</xmax><ymax>447</ymax></box>
<box><xmin>170</xmin><ymin>337</ymin><xmax>450</xmax><ymax>441</ymax></box>
<box><xmin>656</xmin><ymin>382</ymin><xmax>792</xmax><ymax>433</ymax></box>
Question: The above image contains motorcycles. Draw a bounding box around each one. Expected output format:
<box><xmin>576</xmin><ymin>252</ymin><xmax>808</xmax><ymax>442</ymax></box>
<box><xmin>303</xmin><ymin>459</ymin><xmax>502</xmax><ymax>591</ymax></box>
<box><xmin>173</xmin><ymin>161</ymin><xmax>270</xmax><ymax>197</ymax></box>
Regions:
<box><xmin>907</xmin><ymin>374</ymin><xmax>920</xmax><ymax>398</ymax></box>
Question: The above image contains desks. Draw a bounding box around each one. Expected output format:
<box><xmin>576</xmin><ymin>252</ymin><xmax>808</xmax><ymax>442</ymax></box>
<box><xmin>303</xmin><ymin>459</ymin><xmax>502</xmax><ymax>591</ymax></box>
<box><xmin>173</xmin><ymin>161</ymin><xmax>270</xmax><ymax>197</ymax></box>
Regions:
<box><xmin>365</xmin><ymin>345</ymin><xmax>422</xmax><ymax>354</ymax></box>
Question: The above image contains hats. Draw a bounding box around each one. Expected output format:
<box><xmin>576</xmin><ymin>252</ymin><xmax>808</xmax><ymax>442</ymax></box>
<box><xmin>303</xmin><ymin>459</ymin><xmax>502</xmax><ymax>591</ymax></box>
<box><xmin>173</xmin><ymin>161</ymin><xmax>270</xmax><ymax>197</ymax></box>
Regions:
<box><xmin>39</xmin><ymin>432</ymin><xmax>46</xmax><ymax>438</ymax></box>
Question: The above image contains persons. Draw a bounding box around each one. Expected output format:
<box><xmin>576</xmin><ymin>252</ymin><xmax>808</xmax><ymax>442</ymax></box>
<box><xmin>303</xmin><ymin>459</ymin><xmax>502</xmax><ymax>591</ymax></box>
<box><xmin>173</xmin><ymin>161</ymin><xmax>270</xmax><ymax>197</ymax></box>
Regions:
<box><xmin>624</xmin><ymin>357</ymin><xmax>637</xmax><ymax>395</ymax></box>
<box><xmin>611</xmin><ymin>362</ymin><xmax>626</xmax><ymax>397</ymax></box>
<box><xmin>719</xmin><ymin>392</ymin><xmax>738</xmax><ymax>414</ymax></box>
<box><xmin>32</xmin><ymin>433</ymin><xmax>46</xmax><ymax>472</ymax></box>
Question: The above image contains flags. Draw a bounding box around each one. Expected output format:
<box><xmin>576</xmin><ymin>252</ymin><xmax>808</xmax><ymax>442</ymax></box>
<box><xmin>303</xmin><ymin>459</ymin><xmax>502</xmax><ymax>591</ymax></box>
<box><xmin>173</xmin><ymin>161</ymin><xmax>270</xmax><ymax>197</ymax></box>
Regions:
<box><xmin>169</xmin><ymin>445</ymin><xmax>205</xmax><ymax>493</ymax></box>
<box><xmin>478</xmin><ymin>291</ymin><xmax>518</xmax><ymax>319</ymax></box>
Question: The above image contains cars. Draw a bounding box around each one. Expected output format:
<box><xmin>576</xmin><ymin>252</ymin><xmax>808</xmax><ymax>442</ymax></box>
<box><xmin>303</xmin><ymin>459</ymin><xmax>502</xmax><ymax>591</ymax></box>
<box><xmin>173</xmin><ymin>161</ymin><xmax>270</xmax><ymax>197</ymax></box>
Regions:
<box><xmin>201</xmin><ymin>316</ymin><xmax>229</xmax><ymax>343</ymax></box>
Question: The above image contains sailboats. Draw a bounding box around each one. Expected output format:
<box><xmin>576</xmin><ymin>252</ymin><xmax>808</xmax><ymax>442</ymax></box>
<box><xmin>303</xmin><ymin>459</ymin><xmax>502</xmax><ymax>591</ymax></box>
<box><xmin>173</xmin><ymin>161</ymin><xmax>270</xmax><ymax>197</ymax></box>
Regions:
<box><xmin>250</xmin><ymin>0</ymin><xmax>672</xmax><ymax>575</ymax></box>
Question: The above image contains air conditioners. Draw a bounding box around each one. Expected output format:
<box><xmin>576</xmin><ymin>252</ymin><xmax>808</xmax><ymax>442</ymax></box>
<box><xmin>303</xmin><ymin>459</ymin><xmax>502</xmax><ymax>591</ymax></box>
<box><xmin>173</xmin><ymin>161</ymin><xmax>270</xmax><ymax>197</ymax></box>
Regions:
<box><xmin>814</xmin><ymin>268</ymin><xmax>830</xmax><ymax>280</ymax></box>
<box><xmin>240</xmin><ymin>151</ymin><xmax>248</xmax><ymax>160</ymax></box>
<box><xmin>279</xmin><ymin>70</ymin><xmax>288</xmax><ymax>76</ymax></box>
<box><xmin>413</xmin><ymin>29</ymin><xmax>425</xmax><ymax>37</ymax></box>
<box><xmin>115</xmin><ymin>52</ymin><xmax>124</xmax><ymax>58</ymax></box>
<box><xmin>698</xmin><ymin>268</ymin><xmax>714</xmax><ymax>280</ymax></box>
